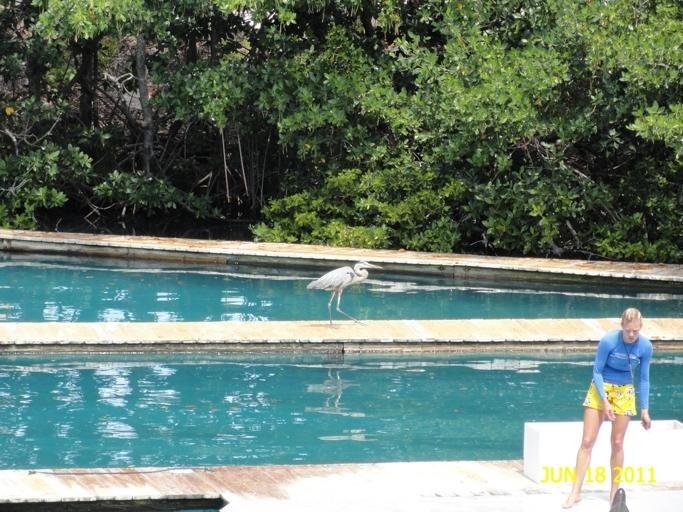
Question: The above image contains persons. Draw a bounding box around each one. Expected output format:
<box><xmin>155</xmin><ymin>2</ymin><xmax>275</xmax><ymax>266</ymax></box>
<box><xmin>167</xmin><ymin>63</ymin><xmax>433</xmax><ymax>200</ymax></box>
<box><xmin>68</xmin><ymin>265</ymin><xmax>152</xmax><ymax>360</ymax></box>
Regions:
<box><xmin>562</xmin><ymin>307</ymin><xmax>653</xmax><ymax>509</ymax></box>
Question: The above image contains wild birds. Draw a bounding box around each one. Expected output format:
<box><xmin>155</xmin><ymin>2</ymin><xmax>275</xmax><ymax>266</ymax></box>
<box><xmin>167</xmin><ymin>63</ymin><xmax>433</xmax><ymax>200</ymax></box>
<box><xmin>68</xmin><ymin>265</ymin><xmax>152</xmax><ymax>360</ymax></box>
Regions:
<box><xmin>306</xmin><ymin>260</ymin><xmax>383</xmax><ymax>325</ymax></box>
